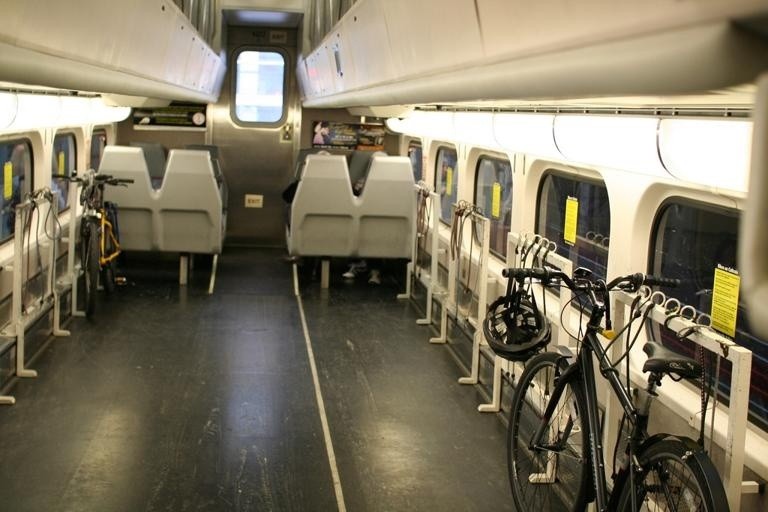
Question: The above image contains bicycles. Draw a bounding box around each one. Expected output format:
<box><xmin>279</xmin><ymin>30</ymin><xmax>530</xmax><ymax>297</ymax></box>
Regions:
<box><xmin>52</xmin><ymin>170</ymin><xmax>135</xmax><ymax>318</ymax></box>
<box><xmin>503</xmin><ymin>267</ymin><xmax>731</xmax><ymax>512</ymax></box>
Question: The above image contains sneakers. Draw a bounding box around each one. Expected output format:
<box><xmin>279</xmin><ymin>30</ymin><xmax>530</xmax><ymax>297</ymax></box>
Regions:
<box><xmin>342</xmin><ymin>262</ymin><xmax>382</xmax><ymax>285</ymax></box>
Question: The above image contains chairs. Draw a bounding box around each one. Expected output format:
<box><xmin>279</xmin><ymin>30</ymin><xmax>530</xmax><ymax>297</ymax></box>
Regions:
<box><xmin>98</xmin><ymin>141</ymin><xmax>228</xmax><ymax>285</ymax></box>
<box><xmin>286</xmin><ymin>149</ymin><xmax>418</xmax><ymax>289</ymax></box>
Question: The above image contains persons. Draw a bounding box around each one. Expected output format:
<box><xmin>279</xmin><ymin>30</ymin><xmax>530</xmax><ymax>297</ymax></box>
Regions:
<box><xmin>283</xmin><ymin>149</ymin><xmax>330</xmax><ymax>281</ymax></box>
<box><xmin>341</xmin><ymin>151</ymin><xmax>389</xmax><ymax>284</ymax></box>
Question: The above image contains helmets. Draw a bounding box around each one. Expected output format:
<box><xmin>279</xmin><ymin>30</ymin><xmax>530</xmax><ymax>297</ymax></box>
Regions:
<box><xmin>483</xmin><ymin>296</ymin><xmax>552</xmax><ymax>361</ymax></box>
